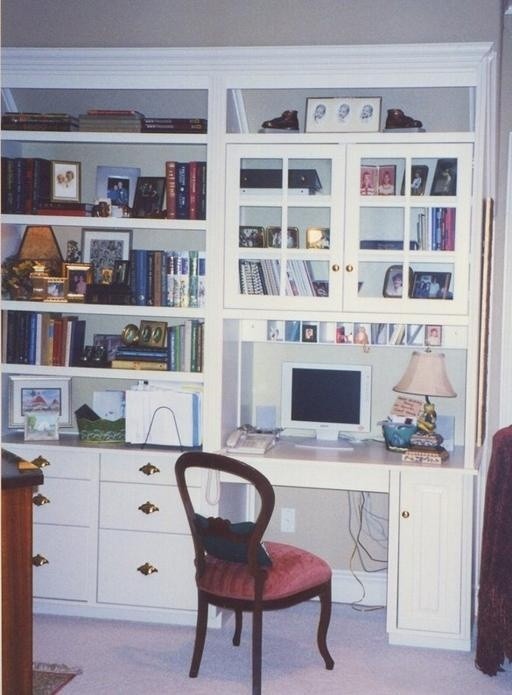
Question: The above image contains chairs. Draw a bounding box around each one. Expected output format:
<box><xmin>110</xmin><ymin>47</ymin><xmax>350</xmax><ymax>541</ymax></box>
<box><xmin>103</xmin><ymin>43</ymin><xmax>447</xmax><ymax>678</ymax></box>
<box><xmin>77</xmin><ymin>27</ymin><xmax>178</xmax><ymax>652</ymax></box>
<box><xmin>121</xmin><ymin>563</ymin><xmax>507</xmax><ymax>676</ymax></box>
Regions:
<box><xmin>176</xmin><ymin>452</ymin><xmax>334</xmax><ymax>694</ymax></box>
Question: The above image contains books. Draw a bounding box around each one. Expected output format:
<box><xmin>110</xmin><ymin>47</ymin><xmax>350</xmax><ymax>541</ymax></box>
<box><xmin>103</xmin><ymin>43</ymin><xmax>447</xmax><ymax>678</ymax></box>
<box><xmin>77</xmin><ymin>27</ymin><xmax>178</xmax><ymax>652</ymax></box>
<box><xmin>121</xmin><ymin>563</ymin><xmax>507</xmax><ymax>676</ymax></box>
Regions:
<box><xmin>416</xmin><ymin>207</ymin><xmax>454</xmax><ymax>250</ymax></box>
<box><xmin>166</xmin><ymin>318</ymin><xmax>203</xmax><ymax>372</ymax></box>
<box><xmin>239</xmin><ymin>259</ymin><xmax>317</xmax><ymax>295</ymax></box>
<box><xmin>131</xmin><ymin>246</ymin><xmax>204</xmax><ymax>308</ymax></box>
<box><xmin>1</xmin><ymin>309</ymin><xmax>85</xmax><ymax>365</ymax></box>
<box><xmin>1</xmin><ymin>104</ymin><xmax>207</xmax><ymax>135</ymax></box>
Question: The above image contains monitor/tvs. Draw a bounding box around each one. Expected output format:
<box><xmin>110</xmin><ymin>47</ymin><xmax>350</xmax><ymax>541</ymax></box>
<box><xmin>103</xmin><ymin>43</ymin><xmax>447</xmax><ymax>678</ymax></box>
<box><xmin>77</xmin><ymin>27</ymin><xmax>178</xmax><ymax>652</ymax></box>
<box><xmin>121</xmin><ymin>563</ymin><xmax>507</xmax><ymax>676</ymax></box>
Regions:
<box><xmin>281</xmin><ymin>362</ymin><xmax>372</xmax><ymax>452</ymax></box>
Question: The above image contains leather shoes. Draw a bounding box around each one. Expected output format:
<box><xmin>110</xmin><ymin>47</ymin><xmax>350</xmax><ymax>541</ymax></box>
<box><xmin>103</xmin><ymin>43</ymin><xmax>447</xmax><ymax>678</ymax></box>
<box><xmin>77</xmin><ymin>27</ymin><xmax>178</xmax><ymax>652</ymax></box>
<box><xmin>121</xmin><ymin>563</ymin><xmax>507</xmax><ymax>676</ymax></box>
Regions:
<box><xmin>385</xmin><ymin>108</ymin><xmax>423</xmax><ymax>129</ymax></box>
<box><xmin>261</xmin><ymin>109</ymin><xmax>299</xmax><ymax>131</ymax></box>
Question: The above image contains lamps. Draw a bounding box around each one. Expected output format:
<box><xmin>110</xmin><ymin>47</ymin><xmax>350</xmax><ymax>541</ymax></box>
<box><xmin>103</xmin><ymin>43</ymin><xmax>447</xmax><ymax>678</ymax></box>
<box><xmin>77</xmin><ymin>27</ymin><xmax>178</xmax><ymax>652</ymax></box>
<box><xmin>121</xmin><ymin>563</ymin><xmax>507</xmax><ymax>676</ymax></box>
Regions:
<box><xmin>392</xmin><ymin>351</ymin><xmax>458</xmax><ymax>466</ymax></box>
<box><xmin>15</xmin><ymin>226</ymin><xmax>63</xmax><ymax>301</ymax></box>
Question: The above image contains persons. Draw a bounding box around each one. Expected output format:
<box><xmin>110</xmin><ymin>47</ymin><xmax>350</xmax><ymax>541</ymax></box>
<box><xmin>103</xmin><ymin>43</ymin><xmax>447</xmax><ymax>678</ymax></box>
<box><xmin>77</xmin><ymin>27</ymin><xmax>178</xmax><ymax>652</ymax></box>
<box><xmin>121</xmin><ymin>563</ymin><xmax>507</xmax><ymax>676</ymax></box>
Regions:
<box><xmin>337</xmin><ymin>104</ymin><xmax>350</xmax><ymax>120</ymax></box>
<box><xmin>359</xmin><ymin>106</ymin><xmax>373</xmax><ymax>120</ymax></box>
<box><xmin>433</xmin><ymin>165</ymin><xmax>452</xmax><ymax>195</ymax></box>
<box><xmin>386</xmin><ymin>273</ymin><xmax>406</xmax><ymax>296</ymax></box>
<box><xmin>428</xmin><ymin>276</ymin><xmax>441</xmax><ymax>297</ymax></box>
<box><xmin>414</xmin><ymin>281</ymin><xmax>424</xmax><ymax>294</ymax></box>
<box><xmin>360</xmin><ymin>171</ymin><xmax>376</xmax><ymax>196</ymax></box>
<box><xmin>412</xmin><ymin>171</ymin><xmax>422</xmax><ymax>194</ymax></box>
<box><xmin>422</xmin><ymin>282</ymin><xmax>431</xmax><ymax>297</ymax></box>
<box><xmin>312</xmin><ymin>104</ymin><xmax>325</xmax><ymax>121</ymax></box>
<box><xmin>379</xmin><ymin>171</ymin><xmax>393</xmax><ymax>195</ymax></box>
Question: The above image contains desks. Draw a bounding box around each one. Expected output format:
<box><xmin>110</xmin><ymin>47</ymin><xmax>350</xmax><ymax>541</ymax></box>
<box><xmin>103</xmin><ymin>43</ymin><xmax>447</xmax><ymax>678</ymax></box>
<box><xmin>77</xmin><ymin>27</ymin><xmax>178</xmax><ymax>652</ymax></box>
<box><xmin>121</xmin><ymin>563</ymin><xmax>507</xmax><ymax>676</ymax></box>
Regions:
<box><xmin>2</xmin><ymin>448</ymin><xmax>43</xmax><ymax>694</ymax></box>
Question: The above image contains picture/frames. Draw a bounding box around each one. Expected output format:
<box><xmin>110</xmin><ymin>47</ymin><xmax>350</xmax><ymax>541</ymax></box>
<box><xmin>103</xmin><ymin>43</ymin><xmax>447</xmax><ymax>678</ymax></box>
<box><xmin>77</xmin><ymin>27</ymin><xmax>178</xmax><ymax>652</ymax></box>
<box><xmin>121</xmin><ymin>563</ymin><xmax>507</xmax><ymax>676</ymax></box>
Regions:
<box><xmin>431</xmin><ymin>160</ymin><xmax>457</xmax><ymax>196</ymax></box>
<box><xmin>413</xmin><ymin>272</ymin><xmax>451</xmax><ymax>299</ymax></box>
<box><xmin>95</xmin><ymin>165</ymin><xmax>140</xmax><ymax>216</ymax></box>
<box><xmin>51</xmin><ymin>159</ymin><xmax>81</xmax><ymax>203</ymax></box>
<box><xmin>383</xmin><ymin>265</ymin><xmax>413</xmax><ymax>299</ymax></box>
<box><xmin>8</xmin><ymin>377</ymin><xmax>71</xmax><ymax>430</ymax></box>
<box><xmin>82</xmin><ymin>229</ymin><xmax>131</xmax><ymax>283</ymax></box>
<box><xmin>425</xmin><ymin>326</ymin><xmax>442</xmax><ymax>345</ymax></box>
<box><xmin>360</xmin><ymin>165</ymin><xmax>378</xmax><ymax>196</ymax></box>
<box><xmin>267</xmin><ymin>227</ymin><xmax>298</xmax><ymax>247</ymax></box>
<box><xmin>401</xmin><ymin>165</ymin><xmax>428</xmax><ymax>196</ymax></box>
<box><xmin>303</xmin><ymin>325</ymin><xmax>316</xmax><ymax>343</ymax></box>
<box><xmin>239</xmin><ymin>226</ymin><xmax>264</xmax><ymax>247</ymax></box>
<box><xmin>379</xmin><ymin>166</ymin><xmax>396</xmax><ymax>195</ymax></box>
<box><xmin>305</xmin><ymin>97</ymin><xmax>381</xmax><ymax>133</ymax></box>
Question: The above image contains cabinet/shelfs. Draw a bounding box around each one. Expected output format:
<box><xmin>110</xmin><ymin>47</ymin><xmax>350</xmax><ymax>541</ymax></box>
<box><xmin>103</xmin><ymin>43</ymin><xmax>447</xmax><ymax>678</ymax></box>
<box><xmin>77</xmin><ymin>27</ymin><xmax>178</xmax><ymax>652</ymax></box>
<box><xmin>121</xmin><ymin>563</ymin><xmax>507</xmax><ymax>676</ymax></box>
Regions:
<box><xmin>1</xmin><ymin>50</ymin><xmax>214</xmax><ymax>625</ymax></box>
<box><xmin>216</xmin><ymin>45</ymin><xmax>496</xmax><ymax>653</ymax></box>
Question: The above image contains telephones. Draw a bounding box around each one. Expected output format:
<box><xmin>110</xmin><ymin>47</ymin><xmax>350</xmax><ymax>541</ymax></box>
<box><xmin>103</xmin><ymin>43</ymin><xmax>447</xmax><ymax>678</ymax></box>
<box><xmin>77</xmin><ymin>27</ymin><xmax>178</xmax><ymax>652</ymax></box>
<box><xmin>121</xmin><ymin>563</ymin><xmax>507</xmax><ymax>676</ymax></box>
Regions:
<box><xmin>226</xmin><ymin>428</ymin><xmax>279</xmax><ymax>456</ymax></box>
